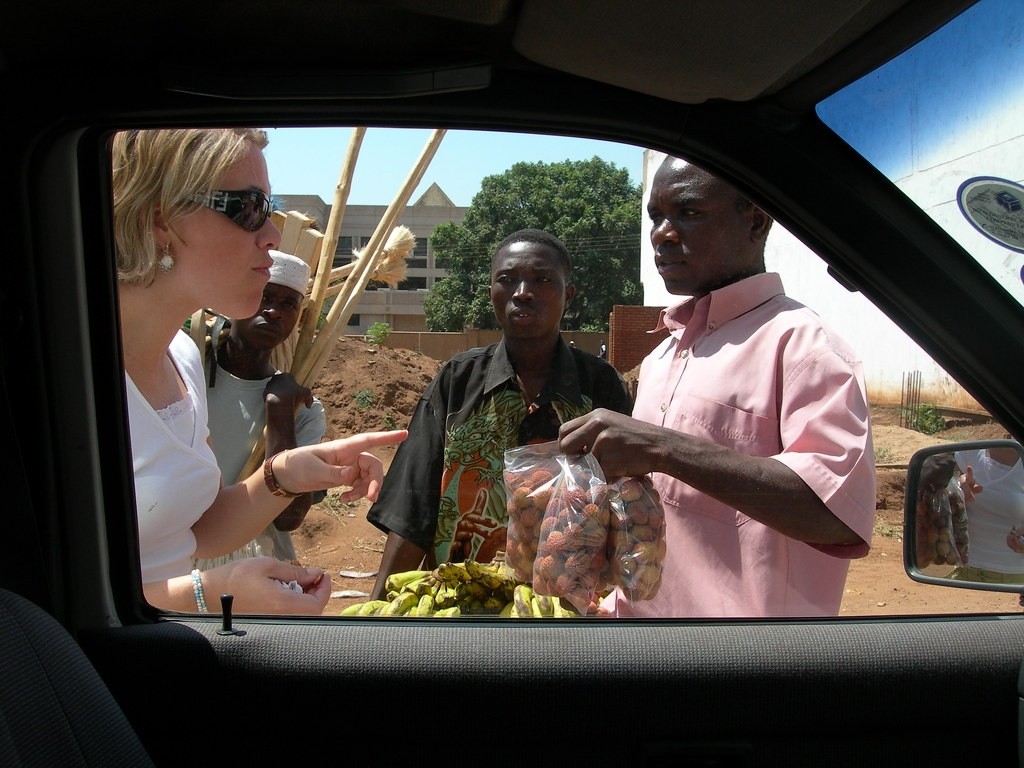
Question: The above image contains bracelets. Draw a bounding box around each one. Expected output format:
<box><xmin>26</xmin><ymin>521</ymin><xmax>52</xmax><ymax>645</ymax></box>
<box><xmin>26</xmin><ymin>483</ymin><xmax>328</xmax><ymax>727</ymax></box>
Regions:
<box><xmin>257</xmin><ymin>447</ymin><xmax>300</xmax><ymax>500</ymax></box>
<box><xmin>184</xmin><ymin>563</ymin><xmax>210</xmax><ymax>619</ymax></box>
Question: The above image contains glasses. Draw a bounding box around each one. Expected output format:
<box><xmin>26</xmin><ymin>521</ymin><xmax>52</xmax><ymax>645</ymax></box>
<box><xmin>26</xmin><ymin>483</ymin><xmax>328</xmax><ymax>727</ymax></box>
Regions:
<box><xmin>183</xmin><ymin>188</ymin><xmax>273</xmax><ymax>232</ymax></box>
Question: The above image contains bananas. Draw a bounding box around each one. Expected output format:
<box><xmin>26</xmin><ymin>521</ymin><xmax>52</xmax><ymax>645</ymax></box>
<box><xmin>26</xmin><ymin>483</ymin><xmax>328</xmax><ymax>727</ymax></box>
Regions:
<box><xmin>339</xmin><ymin>552</ymin><xmax>582</xmax><ymax>618</ymax></box>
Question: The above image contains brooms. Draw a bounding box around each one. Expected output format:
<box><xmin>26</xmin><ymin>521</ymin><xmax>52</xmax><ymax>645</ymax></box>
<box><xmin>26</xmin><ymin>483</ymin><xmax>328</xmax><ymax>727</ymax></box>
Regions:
<box><xmin>190</xmin><ymin>207</ymin><xmax>417</xmax><ymax>373</ymax></box>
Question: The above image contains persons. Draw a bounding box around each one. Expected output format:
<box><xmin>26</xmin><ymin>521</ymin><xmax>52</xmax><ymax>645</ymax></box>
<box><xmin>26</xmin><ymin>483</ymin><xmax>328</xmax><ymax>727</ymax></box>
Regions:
<box><xmin>111</xmin><ymin>127</ymin><xmax>412</xmax><ymax>615</ymax></box>
<box><xmin>915</xmin><ymin>456</ymin><xmax>957</xmax><ymax>494</ymax></box>
<box><xmin>189</xmin><ymin>248</ymin><xmax>325</xmax><ymax>573</ymax></box>
<box><xmin>559</xmin><ymin>155</ymin><xmax>875</xmax><ymax>617</ymax></box>
<box><xmin>369</xmin><ymin>229</ymin><xmax>629</xmax><ymax>604</ymax></box>
<box><xmin>954</xmin><ymin>449</ymin><xmax>1024</xmax><ymax>586</ymax></box>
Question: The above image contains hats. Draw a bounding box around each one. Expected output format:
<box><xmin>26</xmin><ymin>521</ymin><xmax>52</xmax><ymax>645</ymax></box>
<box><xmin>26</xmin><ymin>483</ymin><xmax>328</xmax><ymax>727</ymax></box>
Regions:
<box><xmin>267</xmin><ymin>248</ymin><xmax>311</xmax><ymax>297</ymax></box>
